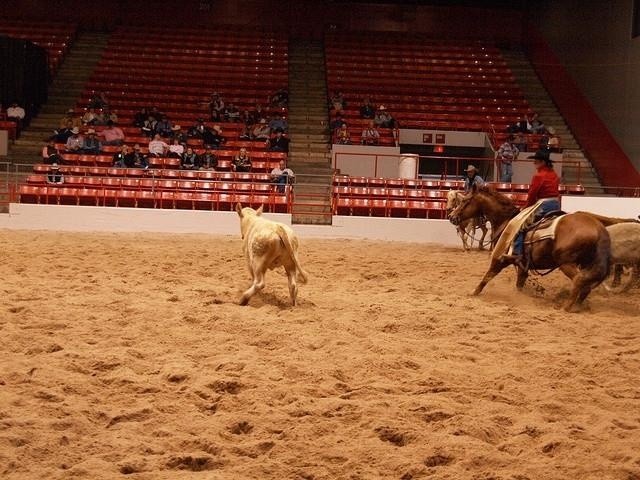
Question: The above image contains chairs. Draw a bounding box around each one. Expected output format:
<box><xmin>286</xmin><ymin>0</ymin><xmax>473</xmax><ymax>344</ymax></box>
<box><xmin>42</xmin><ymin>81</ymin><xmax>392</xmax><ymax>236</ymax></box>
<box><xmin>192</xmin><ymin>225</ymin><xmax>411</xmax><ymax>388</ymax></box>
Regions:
<box><xmin>14</xmin><ymin>2</ymin><xmax>295</xmax><ymax>218</ymax></box>
<box><xmin>321</xmin><ymin>2</ymin><xmax>585</xmax><ymax>218</ymax></box>
<box><xmin>2</xmin><ymin>1</ymin><xmax>83</xmax><ymax>147</ymax></box>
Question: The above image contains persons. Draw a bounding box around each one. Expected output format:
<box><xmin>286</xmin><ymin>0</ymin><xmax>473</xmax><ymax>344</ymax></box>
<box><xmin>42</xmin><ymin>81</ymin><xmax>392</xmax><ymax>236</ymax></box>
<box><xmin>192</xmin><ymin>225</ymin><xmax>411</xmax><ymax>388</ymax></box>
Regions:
<box><xmin>113</xmin><ymin>144</ymin><xmax>149</xmax><ymax>168</ymax></box>
<box><xmin>47</xmin><ymin>163</ymin><xmax>64</xmax><ymax>184</ymax></box>
<box><xmin>495</xmin><ymin>134</ymin><xmax>520</xmax><ymax>185</ymax></box>
<box><xmin>330</xmin><ymin>115</ymin><xmax>351</xmax><ymax>144</ymax></box>
<box><xmin>42</xmin><ymin>140</ymin><xmax>65</xmax><ymax>164</ymax></box>
<box><xmin>133</xmin><ymin>107</ymin><xmax>221</xmax><ymax>171</ymax></box>
<box><xmin>271</xmin><ymin>159</ymin><xmax>294</xmax><ymax>191</ymax></box>
<box><xmin>526</xmin><ymin>112</ymin><xmax>544</xmax><ymax>135</ymax></box>
<box><xmin>360</xmin><ymin>99</ymin><xmax>393</xmax><ymax>128</ymax></box>
<box><xmin>331</xmin><ymin>91</ymin><xmax>344</xmax><ymax>111</ymax></box>
<box><xmin>53</xmin><ymin>88</ymin><xmax>125</xmax><ymax>154</ymax></box>
<box><xmin>461</xmin><ymin>165</ymin><xmax>486</xmax><ymax>196</ymax></box>
<box><xmin>243</xmin><ymin>104</ymin><xmax>289</xmax><ymax>152</ymax></box>
<box><xmin>492</xmin><ymin>146</ymin><xmax>562</xmax><ymax>263</ymax></box>
<box><xmin>208</xmin><ymin>91</ymin><xmax>241</xmax><ymax>123</ymax></box>
<box><xmin>361</xmin><ymin>121</ymin><xmax>380</xmax><ymax>145</ymax></box>
<box><xmin>270</xmin><ymin>88</ymin><xmax>288</xmax><ymax>107</ymax></box>
<box><xmin>231</xmin><ymin>148</ymin><xmax>252</xmax><ymax>172</ymax></box>
<box><xmin>7</xmin><ymin>102</ymin><xmax>25</xmax><ymax>131</ymax></box>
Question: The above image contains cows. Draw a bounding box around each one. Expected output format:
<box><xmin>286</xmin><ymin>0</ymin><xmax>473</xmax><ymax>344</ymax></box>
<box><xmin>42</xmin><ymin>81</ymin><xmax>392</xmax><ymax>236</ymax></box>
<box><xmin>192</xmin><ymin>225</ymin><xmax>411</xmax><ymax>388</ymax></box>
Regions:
<box><xmin>574</xmin><ymin>211</ymin><xmax>640</xmax><ymax>293</ymax></box>
<box><xmin>235</xmin><ymin>201</ymin><xmax>308</xmax><ymax>307</ymax></box>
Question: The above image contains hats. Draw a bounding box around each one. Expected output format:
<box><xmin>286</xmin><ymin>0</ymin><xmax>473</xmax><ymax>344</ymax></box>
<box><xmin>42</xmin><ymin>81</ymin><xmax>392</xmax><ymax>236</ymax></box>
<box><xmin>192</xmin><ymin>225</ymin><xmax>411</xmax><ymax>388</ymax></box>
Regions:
<box><xmin>378</xmin><ymin>105</ymin><xmax>387</xmax><ymax>110</ymax></box>
<box><xmin>464</xmin><ymin>165</ymin><xmax>479</xmax><ymax>173</ymax></box>
<box><xmin>120</xmin><ymin>145</ymin><xmax>128</xmax><ymax>149</ymax></box>
<box><xmin>272</xmin><ymin>128</ymin><xmax>284</xmax><ymax>133</ymax></box>
<box><xmin>132</xmin><ymin>144</ymin><xmax>141</xmax><ymax>150</ymax></box>
<box><xmin>258</xmin><ymin>118</ymin><xmax>268</xmax><ymax>124</ymax></box>
<box><xmin>527</xmin><ymin>150</ymin><xmax>555</xmax><ymax>163</ymax></box>
<box><xmin>50</xmin><ymin>163</ymin><xmax>60</xmax><ymax>169</ymax></box>
<box><xmin>171</xmin><ymin>124</ymin><xmax>183</xmax><ymax>130</ymax></box>
<box><xmin>70</xmin><ymin>127</ymin><xmax>80</xmax><ymax>134</ymax></box>
<box><xmin>84</xmin><ymin>129</ymin><xmax>96</xmax><ymax>135</ymax></box>
<box><xmin>212</xmin><ymin>125</ymin><xmax>220</xmax><ymax>132</ymax></box>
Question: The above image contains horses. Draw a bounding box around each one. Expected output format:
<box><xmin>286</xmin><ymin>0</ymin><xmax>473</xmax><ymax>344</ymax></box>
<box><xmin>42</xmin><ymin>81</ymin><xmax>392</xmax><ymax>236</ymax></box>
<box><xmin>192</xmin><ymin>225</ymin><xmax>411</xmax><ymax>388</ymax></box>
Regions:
<box><xmin>446</xmin><ymin>181</ymin><xmax>611</xmax><ymax>312</ymax></box>
